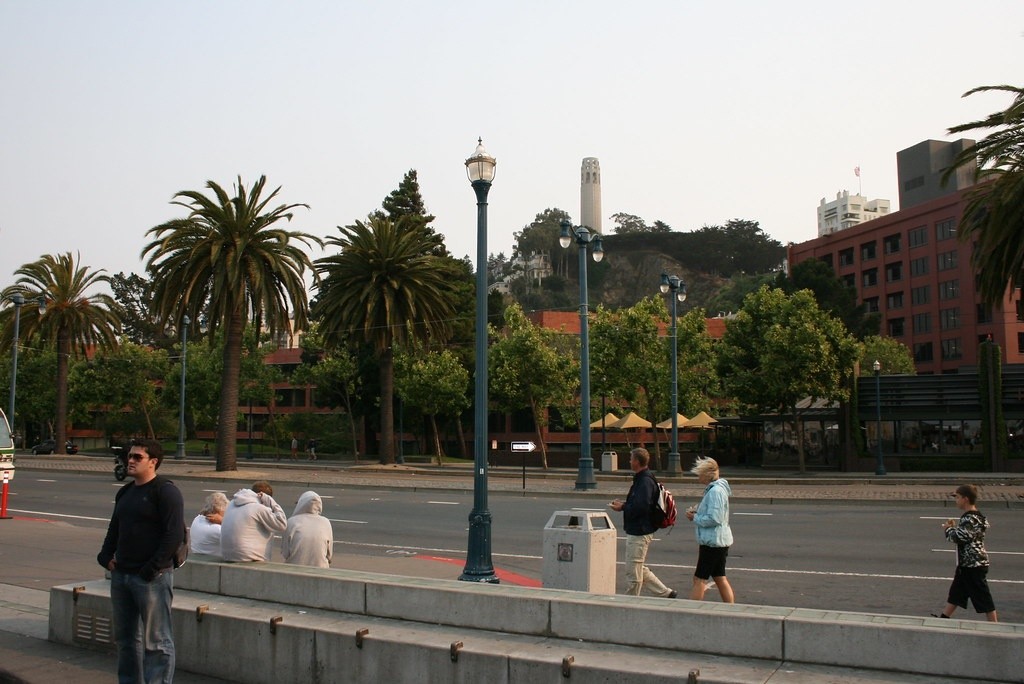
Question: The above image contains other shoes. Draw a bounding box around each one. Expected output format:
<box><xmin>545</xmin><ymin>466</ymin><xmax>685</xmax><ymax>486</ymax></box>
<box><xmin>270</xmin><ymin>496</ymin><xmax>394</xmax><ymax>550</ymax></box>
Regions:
<box><xmin>669</xmin><ymin>590</ymin><xmax>678</xmax><ymax>599</ymax></box>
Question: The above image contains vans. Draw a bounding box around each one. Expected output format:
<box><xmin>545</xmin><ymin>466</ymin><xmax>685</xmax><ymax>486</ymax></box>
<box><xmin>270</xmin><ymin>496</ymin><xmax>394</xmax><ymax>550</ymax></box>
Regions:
<box><xmin>0</xmin><ymin>408</ymin><xmax>15</xmax><ymax>482</ymax></box>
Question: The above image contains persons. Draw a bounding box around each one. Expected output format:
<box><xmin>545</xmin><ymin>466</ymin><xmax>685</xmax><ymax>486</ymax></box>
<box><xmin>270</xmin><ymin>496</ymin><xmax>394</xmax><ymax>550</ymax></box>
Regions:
<box><xmin>221</xmin><ymin>482</ymin><xmax>287</xmax><ymax>562</ymax></box>
<box><xmin>290</xmin><ymin>436</ymin><xmax>317</xmax><ymax>463</ymax></box>
<box><xmin>611</xmin><ymin>448</ymin><xmax>678</xmax><ymax>598</ymax></box>
<box><xmin>97</xmin><ymin>438</ymin><xmax>184</xmax><ymax>684</ymax></box>
<box><xmin>921</xmin><ymin>434</ymin><xmax>941</xmax><ymax>454</ymax></box>
<box><xmin>188</xmin><ymin>493</ymin><xmax>231</xmax><ymax>557</ymax></box>
<box><xmin>33</xmin><ymin>436</ymin><xmax>41</xmax><ymax>446</ymax></box>
<box><xmin>281</xmin><ymin>492</ymin><xmax>333</xmax><ymax>568</ymax></box>
<box><xmin>685</xmin><ymin>455</ymin><xmax>735</xmax><ymax>604</ymax></box>
<box><xmin>968</xmin><ymin>437</ymin><xmax>977</xmax><ymax>452</ymax></box>
<box><xmin>931</xmin><ymin>484</ymin><xmax>997</xmax><ymax>622</ymax></box>
<box><xmin>120</xmin><ymin>438</ymin><xmax>136</xmax><ymax>471</ymax></box>
<box><xmin>757</xmin><ymin>439</ymin><xmax>764</xmax><ymax>452</ymax></box>
<box><xmin>201</xmin><ymin>443</ymin><xmax>209</xmax><ymax>456</ymax></box>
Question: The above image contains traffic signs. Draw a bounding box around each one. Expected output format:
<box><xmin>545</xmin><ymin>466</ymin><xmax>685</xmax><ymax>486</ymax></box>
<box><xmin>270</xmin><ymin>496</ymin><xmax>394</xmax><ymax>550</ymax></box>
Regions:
<box><xmin>512</xmin><ymin>442</ymin><xmax>537</xmax><ymax>452</ymax></box>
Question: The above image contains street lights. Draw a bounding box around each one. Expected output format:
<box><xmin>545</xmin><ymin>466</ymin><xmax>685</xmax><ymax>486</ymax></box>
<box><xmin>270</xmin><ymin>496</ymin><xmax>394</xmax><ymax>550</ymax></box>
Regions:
<box><xmin>0</xmin><ymin>291</ymin><xmax>47</xmax><ymax>435</ymax></box>
<box><xmin>458</xmin><ymin>136</ymin><xmax>502</xmax><ymax>587</ymax></box>
<box><xmin>873</xmin><ymin>360</ymin><xmax>886</xmax><ymax>475</ymax></box>
<box><xmin>558</xmin><ymin>220</ymin><xmax>605</xmax><ymax>489</ymax></box>
<box><xmin>659</xmin><ymin>272</ymin><xmax>687</xmax><ymax>476</ymax></box>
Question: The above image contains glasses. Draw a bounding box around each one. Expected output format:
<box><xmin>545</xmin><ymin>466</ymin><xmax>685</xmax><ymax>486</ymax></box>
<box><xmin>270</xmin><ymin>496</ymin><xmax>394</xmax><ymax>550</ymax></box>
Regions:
<box><xmin>126</xmin><ymin>453</ymin><xmax>148</xmax><ymax>461</ymax></box>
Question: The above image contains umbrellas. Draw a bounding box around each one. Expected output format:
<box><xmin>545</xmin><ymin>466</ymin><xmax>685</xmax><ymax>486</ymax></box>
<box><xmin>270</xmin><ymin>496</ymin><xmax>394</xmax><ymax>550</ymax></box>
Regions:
<box><xmin>827</xmin><ymin>424</ymin><xmax>867</xmax><ymax>429</ymax></box>
<box><xmin>590</xmin><ymin>412</ymin><xmax>718</xmax><ymax>454</ymax></box>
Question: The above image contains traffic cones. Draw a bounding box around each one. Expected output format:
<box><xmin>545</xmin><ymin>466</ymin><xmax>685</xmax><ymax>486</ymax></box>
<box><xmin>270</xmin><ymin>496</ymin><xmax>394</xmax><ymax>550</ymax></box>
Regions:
<box><xmin>1</xmin><ymin>471</ymin><xmax>9</xmax><ymax>518</ymax></box>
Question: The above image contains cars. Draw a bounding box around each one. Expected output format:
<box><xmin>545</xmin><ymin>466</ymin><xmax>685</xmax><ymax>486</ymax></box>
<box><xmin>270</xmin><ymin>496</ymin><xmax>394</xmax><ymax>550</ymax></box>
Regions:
<box><xmin>31</xmin><ymin>439</ymin><xmax>78</xmax><ymax>455</ymax></box>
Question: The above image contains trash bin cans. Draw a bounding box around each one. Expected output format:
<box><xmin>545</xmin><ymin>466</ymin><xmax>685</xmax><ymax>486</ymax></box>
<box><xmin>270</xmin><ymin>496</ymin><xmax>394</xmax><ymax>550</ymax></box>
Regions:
<box><xmin>602</xmin><ymin>451</ymin><xmax>617</xmax><ymax>472</ymax></box>
<box><xmin>542</xmin><ymin>507</ymin><xmax>618</xmax><ymax>594</ymax></box>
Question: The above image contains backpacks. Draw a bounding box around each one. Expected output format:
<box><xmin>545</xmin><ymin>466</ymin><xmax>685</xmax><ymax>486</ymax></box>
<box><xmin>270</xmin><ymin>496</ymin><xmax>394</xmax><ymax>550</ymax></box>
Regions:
<box><xmin>156</xmin><ymin>478</ymin><xmax>189</xmax><ymax>570</ymax></box>
<box><xmin>639</xmin><ymin>471</ymin><xmax>677</xmax><ymax>529</ymax></box>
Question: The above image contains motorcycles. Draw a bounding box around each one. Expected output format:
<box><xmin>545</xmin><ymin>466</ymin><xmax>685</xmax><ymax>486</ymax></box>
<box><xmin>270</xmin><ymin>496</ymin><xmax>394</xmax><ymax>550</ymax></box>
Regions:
<box><xmin>110</xmin><ymin>440</ymin><xmax>135</xmax><ymax>481</ymax></box>
